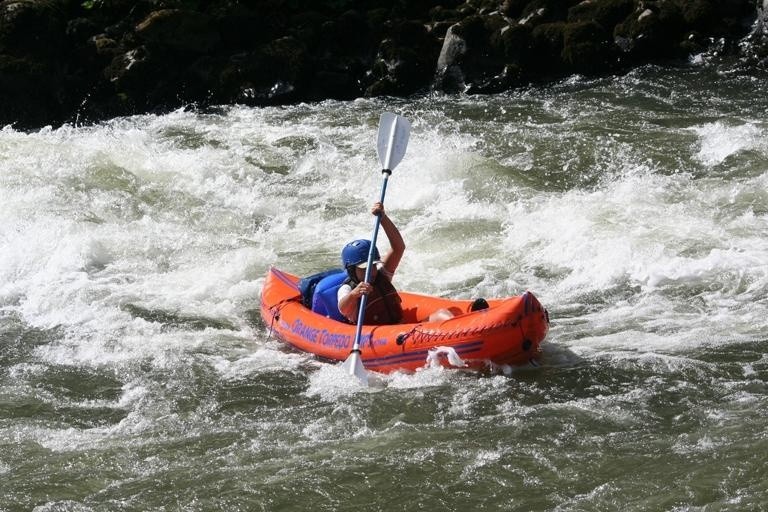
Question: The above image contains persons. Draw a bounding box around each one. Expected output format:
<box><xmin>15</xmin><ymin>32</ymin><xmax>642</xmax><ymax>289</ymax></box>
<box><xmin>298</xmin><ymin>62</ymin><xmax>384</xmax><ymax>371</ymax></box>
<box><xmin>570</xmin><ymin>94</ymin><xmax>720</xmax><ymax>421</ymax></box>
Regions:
<box><xmin>336</xmin><ymin>200</ymin><xmax>490</xmax><ymax>326</ymax></box>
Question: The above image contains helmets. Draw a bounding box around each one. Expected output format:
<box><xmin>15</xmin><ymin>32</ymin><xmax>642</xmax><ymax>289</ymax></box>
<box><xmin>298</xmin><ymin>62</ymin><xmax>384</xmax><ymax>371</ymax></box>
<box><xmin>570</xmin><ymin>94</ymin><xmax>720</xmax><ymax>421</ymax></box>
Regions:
<box><xmin>341</xmin><ymin>239</ymin><xmax>380</xmax><ymax>267</ymax></box>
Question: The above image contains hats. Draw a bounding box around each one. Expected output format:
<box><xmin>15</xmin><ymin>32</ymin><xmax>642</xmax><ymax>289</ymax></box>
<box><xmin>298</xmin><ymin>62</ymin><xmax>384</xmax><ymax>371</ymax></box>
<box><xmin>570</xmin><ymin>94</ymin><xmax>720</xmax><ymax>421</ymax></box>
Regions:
<box><xmin>356</xmin><ymin>260</ymin><xmax>384</xmax><ymax>269</ymax></box>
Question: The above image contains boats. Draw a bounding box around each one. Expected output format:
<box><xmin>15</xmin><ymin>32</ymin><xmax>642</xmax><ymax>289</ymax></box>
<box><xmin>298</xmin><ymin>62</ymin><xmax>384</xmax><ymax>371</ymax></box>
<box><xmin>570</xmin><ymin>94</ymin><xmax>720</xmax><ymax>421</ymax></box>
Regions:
<box><xmin>260</xmin><ymin>266</ymin><xmax>549</xmax><ymax>374</ymax></box>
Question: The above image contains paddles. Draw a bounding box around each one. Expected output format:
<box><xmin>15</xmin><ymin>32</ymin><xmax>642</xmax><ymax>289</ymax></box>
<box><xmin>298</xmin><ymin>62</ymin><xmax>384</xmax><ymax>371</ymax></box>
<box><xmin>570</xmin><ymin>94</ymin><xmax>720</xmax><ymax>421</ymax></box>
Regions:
<box><xmin>340</xmin><ymin>112</ymin><xmax>410</xmax><ymax>384</ymax></box>
